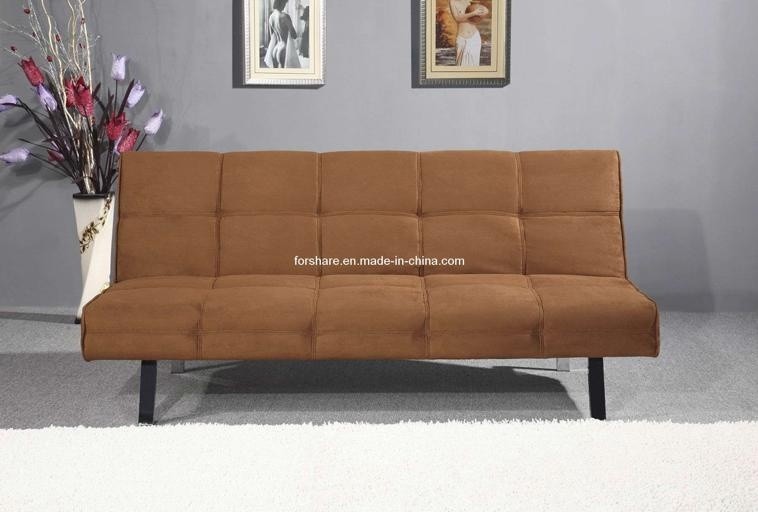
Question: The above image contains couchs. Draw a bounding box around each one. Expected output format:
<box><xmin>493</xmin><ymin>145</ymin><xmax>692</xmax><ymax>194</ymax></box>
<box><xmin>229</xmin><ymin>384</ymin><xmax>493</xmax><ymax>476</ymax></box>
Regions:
<box><xmin>78</xmin><ymin>148</ymin><xmax>660</xmax><ymax>427</ymax></box>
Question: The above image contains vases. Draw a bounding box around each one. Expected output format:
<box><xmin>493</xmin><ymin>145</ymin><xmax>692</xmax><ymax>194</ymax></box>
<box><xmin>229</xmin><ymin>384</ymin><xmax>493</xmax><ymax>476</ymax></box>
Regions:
<box><xmin>72</xmin><ymin>192</ymin><xmax>116</xmax><ymax>324</ymax></box>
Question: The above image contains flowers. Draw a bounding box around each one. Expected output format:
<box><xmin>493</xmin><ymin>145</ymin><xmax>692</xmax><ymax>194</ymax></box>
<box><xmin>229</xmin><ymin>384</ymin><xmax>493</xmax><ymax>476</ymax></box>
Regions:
<box><xmin>0</xmin><ymin>0</ymin><xmax>165</xmax><ymax>195</ymax></box>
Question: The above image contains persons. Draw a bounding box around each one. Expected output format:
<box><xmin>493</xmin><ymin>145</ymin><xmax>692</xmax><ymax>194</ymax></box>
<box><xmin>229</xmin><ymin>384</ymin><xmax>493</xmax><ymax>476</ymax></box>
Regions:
<box><xmin>268</xmin><ymin>0</ymin><xmax>297</xmax><ymax>68</ymax></box>
<box><xmin>450</xmin><ymin>0</ymin><xmax>485</xmax><ymax>67</ymax></box>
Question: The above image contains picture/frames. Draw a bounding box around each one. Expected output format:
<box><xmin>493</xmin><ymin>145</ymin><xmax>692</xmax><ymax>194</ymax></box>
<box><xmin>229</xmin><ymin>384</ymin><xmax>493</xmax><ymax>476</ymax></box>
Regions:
<box><xmin>410</xmin><ymin>0</ymin><xmax>512</xmax><ymax>88</ymax></box>
<box><xmin>231</xmin><ymin>0</ymin><xmax>325</xmax><ymax>89</ymax></box>
